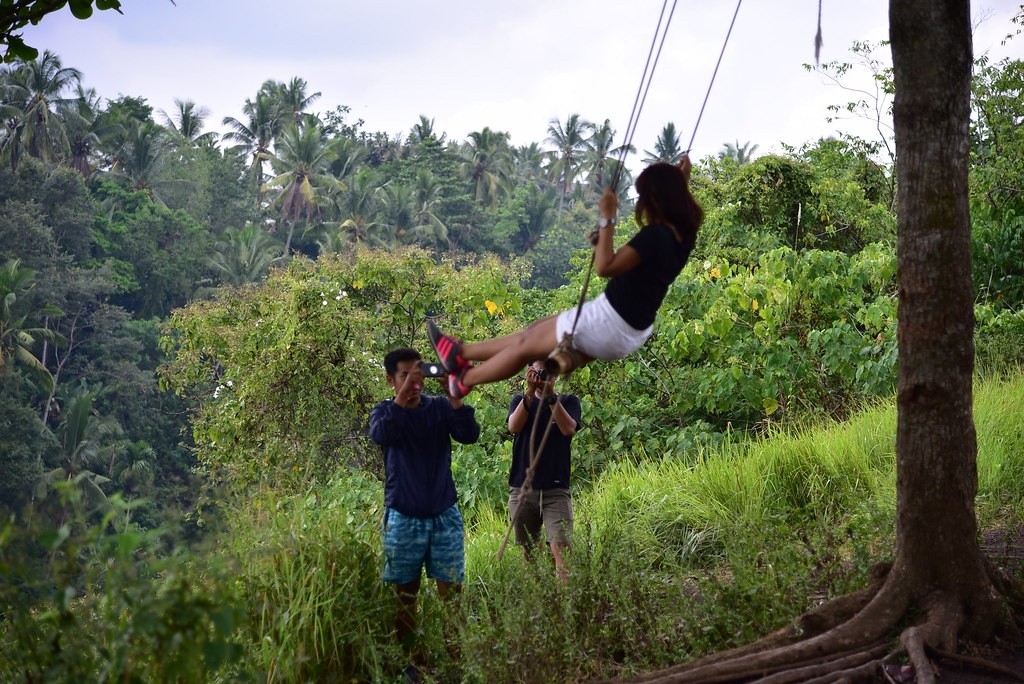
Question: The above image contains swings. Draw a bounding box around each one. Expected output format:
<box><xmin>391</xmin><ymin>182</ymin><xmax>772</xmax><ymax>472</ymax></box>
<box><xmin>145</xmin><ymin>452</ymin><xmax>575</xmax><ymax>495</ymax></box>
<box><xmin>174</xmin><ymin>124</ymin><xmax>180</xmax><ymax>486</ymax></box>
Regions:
<box><xmin>527</xmin><ymin>0</ymin><xmax>745</xmax><ymax>386</ymax></box>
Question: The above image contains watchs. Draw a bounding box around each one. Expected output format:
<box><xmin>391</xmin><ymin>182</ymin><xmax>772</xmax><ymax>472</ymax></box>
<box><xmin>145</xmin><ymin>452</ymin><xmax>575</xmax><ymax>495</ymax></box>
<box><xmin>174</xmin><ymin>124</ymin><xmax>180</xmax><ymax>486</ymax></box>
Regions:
<box><xmin>599</xmin><ymin>218</ymin><xmax>616</xmax><ymax>227</ymax></box>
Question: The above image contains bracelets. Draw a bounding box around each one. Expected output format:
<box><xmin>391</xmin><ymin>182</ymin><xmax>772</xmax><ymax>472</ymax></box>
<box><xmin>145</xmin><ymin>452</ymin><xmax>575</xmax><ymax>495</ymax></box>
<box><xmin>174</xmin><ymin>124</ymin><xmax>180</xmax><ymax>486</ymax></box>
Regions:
<box><xmin>399</xmin><ymin>391</ymin><xmax>410</xmax><ymax>400</ymax></box>
<box><xmin>545</xmin><ymin>394</ymin><xmax>558</xmax><ymax>404</ymax></box>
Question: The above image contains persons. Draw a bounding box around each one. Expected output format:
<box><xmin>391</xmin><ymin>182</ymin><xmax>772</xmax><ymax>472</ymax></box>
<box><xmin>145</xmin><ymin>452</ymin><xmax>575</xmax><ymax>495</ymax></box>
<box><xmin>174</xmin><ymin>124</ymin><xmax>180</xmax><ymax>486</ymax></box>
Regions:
<box><xmin>506</xmin><ymin>360</ymin><xmax>581</xmax><ymax>586</ymax></box>
<box><xmin>370</xmin><ymin>349</ymin><xmax>480</xmax><ymax>663</ymax></box>
<box><xmin>428</xmin><ymin>154</ymin><xmax>704</xmax><ymax>397</ymax></box>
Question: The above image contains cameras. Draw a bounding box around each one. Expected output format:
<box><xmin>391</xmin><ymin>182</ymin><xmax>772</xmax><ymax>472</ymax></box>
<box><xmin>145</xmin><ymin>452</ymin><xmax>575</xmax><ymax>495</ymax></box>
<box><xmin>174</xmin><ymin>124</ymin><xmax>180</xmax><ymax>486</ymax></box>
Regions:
<box><xmin>536</xmin><ymin>369</ymin><xmax>549</xmax><ymax>380</ymax></box>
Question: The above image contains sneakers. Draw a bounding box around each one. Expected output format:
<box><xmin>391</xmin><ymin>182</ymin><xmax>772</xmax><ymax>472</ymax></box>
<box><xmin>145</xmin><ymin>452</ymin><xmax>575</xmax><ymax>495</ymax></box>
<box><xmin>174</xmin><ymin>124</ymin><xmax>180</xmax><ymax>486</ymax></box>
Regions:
<box><xmin>426</xmin><ymin>319</ymin><xmax>469</xmax><ymax>373</ymax></box>
<box><xmin>447</xmin><ymin>364</ymin><xmax>475</xmax><ymax>399</ymax></box>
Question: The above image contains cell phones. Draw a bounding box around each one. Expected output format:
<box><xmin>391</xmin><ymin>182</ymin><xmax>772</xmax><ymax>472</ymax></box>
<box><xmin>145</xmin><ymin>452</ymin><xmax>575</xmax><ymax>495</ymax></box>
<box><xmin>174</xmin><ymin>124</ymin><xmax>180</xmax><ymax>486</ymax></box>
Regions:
<box><xmin>419</xmin><ymin>363</ymin><xmax>445</xmax><ymax>377</ymax></box>
